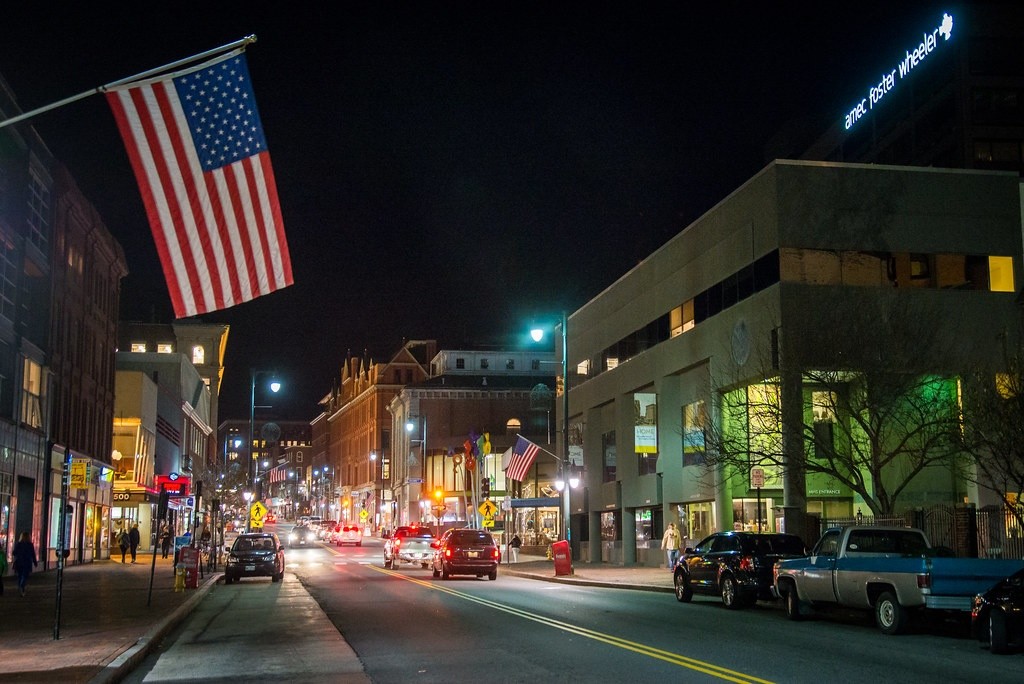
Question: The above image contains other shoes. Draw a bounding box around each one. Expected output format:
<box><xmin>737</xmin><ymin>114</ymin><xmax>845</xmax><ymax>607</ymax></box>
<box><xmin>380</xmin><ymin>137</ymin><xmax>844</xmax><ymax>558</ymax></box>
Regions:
<box><xmin>130</xmin><ymin>559</ymin><xmax>136</xmax><ymax>564</ymax></box>
<box><xmin>20</xmin><ymin>592</ymin><xmax>25</xmax><ymax>597</ymax></box>
<box><xmin>122</xmin><ymin>561</ymin><xmax>126</xmax><ymax>565</ymax></box>
<box><xmin>671</xmin><ymin>569</ymin><xmax>674</xmax><ymax>573</ymax></box>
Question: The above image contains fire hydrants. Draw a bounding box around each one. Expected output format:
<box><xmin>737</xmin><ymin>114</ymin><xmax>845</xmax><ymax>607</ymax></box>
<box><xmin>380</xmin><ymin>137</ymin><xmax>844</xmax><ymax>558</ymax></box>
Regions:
<box><xmin>545</xmin><ymin>544</ymin><xmax>554</xmax><ymax>562</ymax></box>
<box><xmin>173</xmin><ymin>563</ymin><xmax>189</xmax><ymax>592</ymax></box>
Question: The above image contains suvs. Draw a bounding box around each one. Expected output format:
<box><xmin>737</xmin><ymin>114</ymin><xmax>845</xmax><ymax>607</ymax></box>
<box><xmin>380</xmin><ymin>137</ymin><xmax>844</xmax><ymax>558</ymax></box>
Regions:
<box><xmin>226</xmin><ymin>532</ymin><xmax>286</xmax><ymax>585</ymax></box>
<box><xmin>384</xmin><ymin>524</ymin><xmax>440</xmax><ymax>570</ymax></box>
<box><xmin>431</xmin><ymin>527</ymin><xmax>498</xmax><ymax>582</ymax></box>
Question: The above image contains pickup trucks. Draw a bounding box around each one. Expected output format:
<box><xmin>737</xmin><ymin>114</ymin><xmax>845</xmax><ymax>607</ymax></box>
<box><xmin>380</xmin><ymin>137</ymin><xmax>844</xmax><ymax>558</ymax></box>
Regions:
<box><xmin>773</xmin><ymin>524</ymin><xmax>1024</xmax><ymax>633</ymax></box>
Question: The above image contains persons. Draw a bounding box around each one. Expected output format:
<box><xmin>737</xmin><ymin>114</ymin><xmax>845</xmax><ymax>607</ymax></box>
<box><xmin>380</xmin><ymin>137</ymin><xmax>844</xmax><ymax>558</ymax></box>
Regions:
<box><xmin>509</xmin><ymin>535</ymin><xmax>521</xmax><ymax>562</ymax></box>
<box><xmin>184</xmin><ymin>528</ymin><xmax>191</xmax><ymax>536</ymax></box>
<box><xmin>11</xmin><ymin>533</ymin><xmax>37</xmax><ymax>597</ymax></box>
<box><xmin>527</xmin><ymin>518</ymin><xmax>533</xmax><ymax>529</ymax></box>
<box><xmin>0</xmin><ymin>543</ymin><xmax>8</xmax><ymax>593</ymax></box>
<box><xmin>159</xmin><ymin>526</ymin><xmax>171</xmax><ymax>559</ymax></box>
<box><xmin>201</xmin><ymin>526</ymin><xmax>211</xmax><ymax>547</ymax></box>
<box><xmin>661</xmin><ymin>523</ymin><xmax>681</xmax><ymax>572</ymax></box>
<box><xmin>118</xmin><ymin>523</ymin><xmax>140</xmax><ymax>563</ymax></box>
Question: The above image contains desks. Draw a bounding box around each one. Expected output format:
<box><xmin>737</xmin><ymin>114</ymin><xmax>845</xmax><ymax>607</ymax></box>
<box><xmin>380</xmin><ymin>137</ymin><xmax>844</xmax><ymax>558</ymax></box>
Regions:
<box><xmin>115</xmin><ymin>472</ymin><xmax>122</xmax><ymax>480</ymax></box>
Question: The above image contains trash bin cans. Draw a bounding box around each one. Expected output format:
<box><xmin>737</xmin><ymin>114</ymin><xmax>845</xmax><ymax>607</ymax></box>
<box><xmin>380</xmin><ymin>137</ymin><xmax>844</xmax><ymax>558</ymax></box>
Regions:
<box><xmin>552</xmin><ymin>540</ymin><xmax>572</xmax><ymax>576</ymax></box>
<box><xmin>179</xmin><ymin>547</ymin><xmax>199</xmax><ymax>589</ymax></box>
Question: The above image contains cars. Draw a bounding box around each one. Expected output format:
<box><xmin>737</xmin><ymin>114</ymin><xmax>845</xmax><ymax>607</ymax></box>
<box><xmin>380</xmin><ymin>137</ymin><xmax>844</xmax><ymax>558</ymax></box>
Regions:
<box><xmin>673</xmin><ymin>530</ymin><xmax>809</xmax><ymax>610</ymax></box>
<box><xmin>971</xmin><ymin>569</ymin><xmax>1024</xmax><ymax>656</ymax></box>
<box><xmin>265</xmin><ymin>515</ymin><xmax>277</xmax><ymax>523</ymax></box>
<box><xmin>289</xmin><ymin>515</ymin><xmax>363</xmax><ymax>547</ymax></box>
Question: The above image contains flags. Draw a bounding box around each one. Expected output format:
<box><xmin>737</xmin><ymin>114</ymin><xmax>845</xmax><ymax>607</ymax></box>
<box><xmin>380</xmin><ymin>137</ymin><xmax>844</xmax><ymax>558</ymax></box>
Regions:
<box><xmin>270</xmin><ymin>464</ymin><xmax>286</xmax><ymax>483</ymax></box>
<box><xmin>310</xmin><ymin>496</ymin><xmax>316</xmax><ymax>506</ymax></box>
<box><xmin>367</xmin><ymin>492</ymin><xmax>374</xmax><ymax>509</ymax></box>
<box><xmin>320</xmin><ymin>495</ymin><xmax>326</xmax><ymax>506</ymax></box>
<box><xmin>506</xmin><ymin>437</ymin><xmax>539</xmax><ymax>482</ymax></box>
<box><xmin>108</xmin><ymin>48</ymin><xmax>295</xmax><ymax>319</ymax></box>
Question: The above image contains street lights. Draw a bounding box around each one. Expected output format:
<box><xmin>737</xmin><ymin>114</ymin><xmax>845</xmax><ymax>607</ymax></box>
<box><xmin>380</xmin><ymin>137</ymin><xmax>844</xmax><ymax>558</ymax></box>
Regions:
<box><xmin>370</xmin><ymin>448</ymin><xmax>385</xmax><ymax>511</ymax></box>
<box><xmin>533</xmin><ymin>309</ymin><xmax>575</xmax><ymax>572</ymax></box>
<box><xmin>289</xmin><ymin>469</ymin><xmax>298</xmax><ymax>512</ymax></box>
<box><xmin>314</xmin><ymin>465</ymin><xmax>328</xmax><ymax>515</ymax></box>
<box><xmin>246</xmin><ymin>369</ymin><xmax>281</xmax><ymax>531</ymax></box>
<box><xmin>407</xmin><ymin>414</ymin><xmax>427</xmax><ymax>525</ymax></box>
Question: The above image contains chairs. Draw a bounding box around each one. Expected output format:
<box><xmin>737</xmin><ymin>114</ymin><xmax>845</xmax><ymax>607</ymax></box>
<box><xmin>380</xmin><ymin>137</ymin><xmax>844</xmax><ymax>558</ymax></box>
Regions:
<box><xmin>241</xmin><ymin>541</ymin><xmax>255</xmax><ymax>550</ymax></box>
<box><xmin>261</xmin><ymin>540</ymin><xmax>271</xmax><ymax>550</ymax></box>
<box><xmin>757</xmin><ymin>538</ymin><xmax>771</xmax><ymax>552</ymax></box>
<box><xmin>417</xmin><ymin>530</ymin><xmax>430</xmax><ymax>535</ymax></box>
<box><xmin>399</xmin><ymin>531</ymin><xmax>406</xmax><ymax>537</ymax></box>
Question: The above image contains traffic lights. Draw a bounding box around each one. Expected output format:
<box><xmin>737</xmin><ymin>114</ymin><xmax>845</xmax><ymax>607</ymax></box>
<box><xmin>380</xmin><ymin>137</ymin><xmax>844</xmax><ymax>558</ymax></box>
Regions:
<box><xmin>345</xmin><ymin>498</ymin><xmax>349</xmax><ymax>508</ymax></box>
<box><xmin>435</xmin><ymin>485</ymin><xmax>443</xmax><ymax>503</ymax></box>
